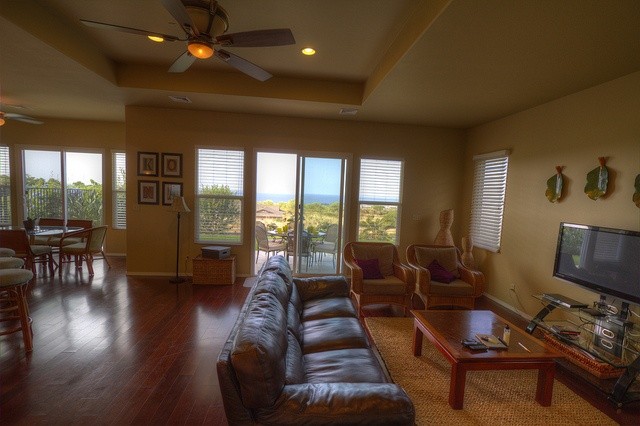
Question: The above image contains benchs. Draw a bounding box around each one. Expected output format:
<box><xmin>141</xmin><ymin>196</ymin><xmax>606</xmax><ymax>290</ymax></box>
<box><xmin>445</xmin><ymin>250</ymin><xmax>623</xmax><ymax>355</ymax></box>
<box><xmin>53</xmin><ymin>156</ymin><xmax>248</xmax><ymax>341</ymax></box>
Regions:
<box><xmin>0</xmin><ymin>229</ymin><xmax>54</xmax><ymax>277</ymax></box>
<box><xmin>31</xmin><ymin>218</ymin><xmax>93</xmax><ymax>247</ymax></box>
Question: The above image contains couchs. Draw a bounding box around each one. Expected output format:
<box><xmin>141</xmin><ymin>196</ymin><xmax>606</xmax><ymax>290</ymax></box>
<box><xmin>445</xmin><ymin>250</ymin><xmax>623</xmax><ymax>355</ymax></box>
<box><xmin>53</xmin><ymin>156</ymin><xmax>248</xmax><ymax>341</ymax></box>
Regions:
<box><xmin>215</xmin><ymin>257</ymin><xmax>415</xmax><ymax>426</ymax></box>
<box><xmin>343</xmin><ymin>243</ymin><xmax>412</xmax><ymax>316</ymax></box>
<box><xmin>406</xmin><ymin>243</ymin><xmax>486</xmax><ymax>310</ymax></box>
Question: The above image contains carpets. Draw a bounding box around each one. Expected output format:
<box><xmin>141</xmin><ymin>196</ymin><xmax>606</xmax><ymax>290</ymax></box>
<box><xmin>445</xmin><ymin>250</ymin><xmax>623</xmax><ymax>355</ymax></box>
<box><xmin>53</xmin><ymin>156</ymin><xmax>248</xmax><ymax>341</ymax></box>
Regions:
<box><xmin>363</xmin><ymin>316</ymin><xmax>621</xmax><ymax>425</ymax></box>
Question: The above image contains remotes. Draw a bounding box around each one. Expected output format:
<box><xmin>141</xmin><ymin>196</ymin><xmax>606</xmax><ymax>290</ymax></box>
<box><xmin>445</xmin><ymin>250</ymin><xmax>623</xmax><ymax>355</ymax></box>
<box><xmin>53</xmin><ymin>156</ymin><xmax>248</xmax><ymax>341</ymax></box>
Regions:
<box><xmin>464</xmin><ymin>341</ymin><xmax>481</xmax><ymax>345</ymax></box>
<box><xmin>469</xmin><ymin>344</ymin><xmax>487</xmax><ymax>351</ymax></box>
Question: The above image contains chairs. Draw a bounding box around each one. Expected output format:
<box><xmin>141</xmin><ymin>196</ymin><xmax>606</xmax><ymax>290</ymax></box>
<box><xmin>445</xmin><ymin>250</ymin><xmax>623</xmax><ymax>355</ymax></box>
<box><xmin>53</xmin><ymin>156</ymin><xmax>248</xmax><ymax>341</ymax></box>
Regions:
<box><xmin>309</xmin><ymin>224</ymin><xmax>338</xmax><ymax>270</ymax></box>
<box><xmin>256</xmin><ymin>221</ymin><xmax>286</xmax><ymax>262</ymax></box>
<box><xmin>286</xmin><ymin>230</ymin><xmax>312</xmax><ymax>272</ymax></box>
<box><xmin>59</xmin><ymin>223</ymin><xmax>112</xmax><ymax>277</ymax></box>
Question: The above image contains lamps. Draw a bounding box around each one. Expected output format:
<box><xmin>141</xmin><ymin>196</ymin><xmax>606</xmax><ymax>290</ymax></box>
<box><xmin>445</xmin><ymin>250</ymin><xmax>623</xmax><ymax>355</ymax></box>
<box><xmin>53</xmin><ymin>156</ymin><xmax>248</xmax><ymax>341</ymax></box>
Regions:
<box><xmin>78</xmin><ymin>1</ymin><xmax>294</xmax><ymax>83</ymax></box>
<box><xmin>165</xmin><ymin>196</ymin><xmax>189</xmax><ymax>283</ymax></box>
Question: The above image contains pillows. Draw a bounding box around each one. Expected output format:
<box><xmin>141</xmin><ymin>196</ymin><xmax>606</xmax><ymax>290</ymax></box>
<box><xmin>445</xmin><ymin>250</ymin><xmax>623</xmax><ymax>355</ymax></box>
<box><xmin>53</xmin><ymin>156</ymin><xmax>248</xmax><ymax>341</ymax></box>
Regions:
<box><xmin>354</xmin><ymin>259</ymin><xmax>384</xmax><ymax>279</ymax></box>
<box><xmin>424</xmin><ymin>259</ymin><xmax>457</xmax><ymax>284</ymax></box>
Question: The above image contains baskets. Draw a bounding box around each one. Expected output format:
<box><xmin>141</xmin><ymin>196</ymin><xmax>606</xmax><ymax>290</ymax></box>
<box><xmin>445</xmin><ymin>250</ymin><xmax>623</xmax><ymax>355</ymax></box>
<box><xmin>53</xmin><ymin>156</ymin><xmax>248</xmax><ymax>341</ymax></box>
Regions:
<box><xmin>543</xmin><ymin>331</ymin><xmax>627</xmax><ymax>379</ymax></box>
<box><xmin>24</xmin><ymin>218</ymin><xmax>40</xmax><ymax>229</ymax></box>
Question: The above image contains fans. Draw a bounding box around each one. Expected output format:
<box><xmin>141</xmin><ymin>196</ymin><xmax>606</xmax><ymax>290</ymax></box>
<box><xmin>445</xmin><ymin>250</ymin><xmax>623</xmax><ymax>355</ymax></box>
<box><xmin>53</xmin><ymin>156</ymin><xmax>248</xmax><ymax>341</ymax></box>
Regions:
<box><xmin>0</xmin><ymin>111</ymin><xmax>46</xmax><ymax>128</ymax></box>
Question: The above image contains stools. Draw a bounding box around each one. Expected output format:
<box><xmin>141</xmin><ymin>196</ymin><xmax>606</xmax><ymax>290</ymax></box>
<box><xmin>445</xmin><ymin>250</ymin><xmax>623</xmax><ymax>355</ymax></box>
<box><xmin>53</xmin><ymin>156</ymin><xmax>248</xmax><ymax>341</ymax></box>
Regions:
<box><xmin>1</xmin><ymin>257</ymin><xmax>25</xmax><ymax>325</ymax></box>
<box><xmin>0</xmin><ymin>247</ymin><xmax>16</xmax><ymax>316</ymax></box>
<box><xmin>0</xmin><ymin>268</ymin><xmax>34</xmax><ymax>351</ymax></box>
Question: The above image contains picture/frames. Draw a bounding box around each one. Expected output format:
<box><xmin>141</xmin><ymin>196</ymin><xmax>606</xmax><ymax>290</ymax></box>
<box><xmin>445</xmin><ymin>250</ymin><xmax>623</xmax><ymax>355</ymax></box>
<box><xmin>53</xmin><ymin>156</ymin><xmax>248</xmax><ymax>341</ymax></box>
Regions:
<box><xmin>162</xmin><ymin>182</ymin><xmax>183</xmax><ymax>207</ymax></box>
<box><xmin>162</xmin><ymin>151</ymin><xmax>183</xmax><ymax>177</ymax></box>
<box><xmin>137</xmin><ymin>151</ymin><xmax>159</xmax><ymax>176</ymax></box>
<box><xmin>137</xmin><ymin>180</ymin><xmax>159</xmax><ymax>204</ymax></box>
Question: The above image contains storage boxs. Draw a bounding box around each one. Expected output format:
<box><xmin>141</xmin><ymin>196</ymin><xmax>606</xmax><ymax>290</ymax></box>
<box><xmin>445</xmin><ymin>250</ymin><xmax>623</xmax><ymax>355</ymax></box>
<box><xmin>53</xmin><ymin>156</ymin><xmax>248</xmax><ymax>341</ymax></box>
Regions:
<box><xmin>193</xmin><ymin>254</ymin><xmax>237</xmax><ymax>284</ymax></box>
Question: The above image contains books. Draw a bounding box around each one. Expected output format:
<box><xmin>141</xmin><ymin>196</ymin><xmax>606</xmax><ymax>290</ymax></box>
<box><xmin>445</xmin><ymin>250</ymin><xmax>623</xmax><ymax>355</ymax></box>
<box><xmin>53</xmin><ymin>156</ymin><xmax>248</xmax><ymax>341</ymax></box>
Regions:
<box><xmin>475</xmin><ymin>334</ymin><xmax>507</xmax><ymax>350</ymax></box>
<box><xmin>551</xmin><ymin>325</ymin><xmax>581</xmax><ymax>335</ymax></box>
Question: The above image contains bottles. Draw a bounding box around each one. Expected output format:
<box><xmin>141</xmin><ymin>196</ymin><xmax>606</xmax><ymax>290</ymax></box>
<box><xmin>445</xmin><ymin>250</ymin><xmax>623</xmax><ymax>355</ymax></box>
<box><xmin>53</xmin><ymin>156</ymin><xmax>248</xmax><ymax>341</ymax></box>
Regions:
<box><xmin>503</xmin><ymin>325</ymin><xmax>511</xmax><ymax>347</ymax></box>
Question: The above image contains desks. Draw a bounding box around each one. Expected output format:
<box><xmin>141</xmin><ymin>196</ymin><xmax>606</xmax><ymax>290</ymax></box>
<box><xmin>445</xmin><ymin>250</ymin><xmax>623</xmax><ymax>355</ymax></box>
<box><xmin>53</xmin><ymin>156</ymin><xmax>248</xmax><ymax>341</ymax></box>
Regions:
<box><xmin>266</xmin><ymin>228</ymin><xmax>325</xmax><ymax>267</ymax></box>
<box><xmin>519</xmin><ymin>292</ymin><xmax>640</xmax><ymax>406</ymax></box>
<box><xmin>18</xmin><ymin>226</ymin><xmax>82</xmax><ymax>267</ymax></box>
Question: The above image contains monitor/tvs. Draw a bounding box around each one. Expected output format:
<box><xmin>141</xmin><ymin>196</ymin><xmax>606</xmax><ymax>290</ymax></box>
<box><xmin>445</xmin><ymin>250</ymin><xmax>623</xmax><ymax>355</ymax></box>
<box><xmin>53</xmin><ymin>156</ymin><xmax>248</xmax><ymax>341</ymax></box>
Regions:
<box><xmin>550</xmin><ymin>222</ymin><xmax>640</xmax><ymax>307</ymax></box>
